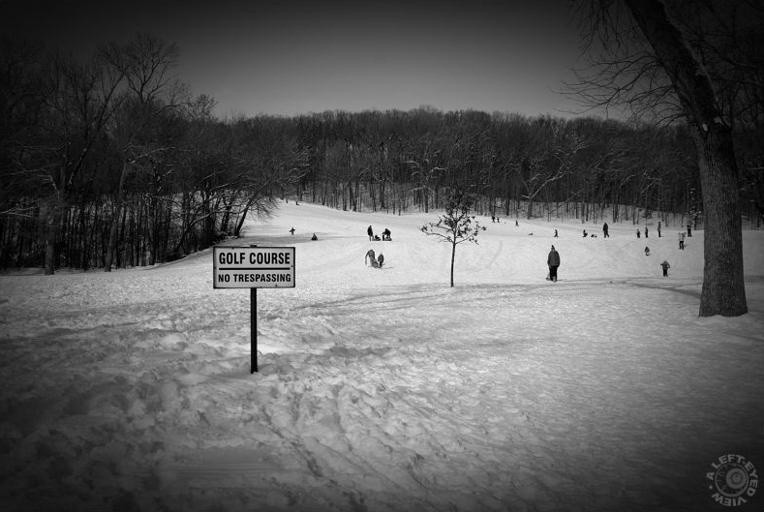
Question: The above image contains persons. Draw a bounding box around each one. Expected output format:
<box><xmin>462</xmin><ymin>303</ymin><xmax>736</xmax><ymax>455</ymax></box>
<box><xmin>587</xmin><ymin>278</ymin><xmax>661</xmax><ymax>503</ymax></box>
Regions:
<box><xmin>367</xmin><ymin>225</ymin><xmax>392</xmax><ymax>241</ymax></box>
<box><xmin>547</xmin><ymin>245</ymin><xmax>560</xmax><ymax>282</ymax></box>
<box><xmin>554</xmin><ymin>230</ymin><xmax>558</xmax><ymax>238</ymax></box>
<box><xmin>582</xmin><ymin>230</ymin><xmax>587</xmax><ymax>237</ymax></box>
<box><xmin>660</xmin><ymin>260</ymin><xmax>670</xmax><ymax>277</ymax></box>
<box><xmin>515</xmin><ymin>220</ymin><xmax>519</xmax><ymax>226</ymax></box>
<box><xmin>365</xmin><ymin>249</ymin><xmax>384</xmax><ymax>268</ymax></box>
<box><xmin>603</xmin><ymin>222</ymin><xmax>609</xmax><ymax>237</ymax></box>
<box><xmin>677</xmin><ymin>220</ymin><xmax>692</xmax><ymax>249</ymax></box>
<box><xmin>290</xmin><ymin>227</ymin><xmax>295</xmax><ymax>235</ymax></box>
<box><xmin>645</xmin><ymin>246</ymin><xmax>649</xmax><ymax>256</ymax></box>
<box><xmin>492</xmin><ymin>216</ymin><xmax>499</xmax><ymax>223</ymax></box>
<box><xmin>312</xmin><ymin>233</ymin><xmax>318</xmax><ymax>240</ymax></box>
<box><xmin>637</xmin><ymin>222</ymin><xmax>661</xmax><ymax>237</ymax></box>
